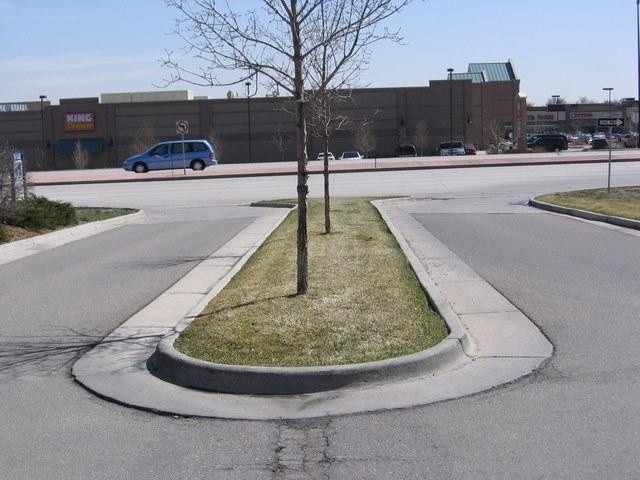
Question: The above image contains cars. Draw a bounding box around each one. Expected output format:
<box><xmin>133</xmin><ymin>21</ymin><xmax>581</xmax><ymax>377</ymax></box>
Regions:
<box><xmin>317</xmin><ymin>151</ymin><xmax>365</xmax><ymax>160</ymax></box>
<box><xmin>439</xmin><ymin>141</ymin><xmax>477</xmax><ymax>155</ymax></box>
<box><xmin>396</xmin><ymin>144</ymin><xmax>418</xmax><ymax>157</ymax></box>
<box><xmin>527</xmin><ymin>134</ymin><xmax>568</xmax><ymax>152</ymax></box>
<box><xmin>591</xmin><ymin>131</ymin><xmax>639</xmax><ymax>148</ymax></box>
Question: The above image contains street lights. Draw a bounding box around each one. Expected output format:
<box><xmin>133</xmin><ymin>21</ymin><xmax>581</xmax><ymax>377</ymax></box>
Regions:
<box><xmin>245</xmin><ymin>82</ymin><xmax>252</xmax><ymax>163</ymax></box>
<box><xmin>602</xmin><ymin>88</ymin><xmax>614</xmax><ymax>118</ymax></box>
<box><xmin>39</xmin><ymin>95</ymin><xmax>47</xmax><ymax>170</ymax></box>
<box><xmin>552</xmin><ymin>95</ymin><xmax>560</xmax><ymax>132</ymax></box>
<box><xmin>447</xmin><ymin>69</ymin><xmax>453</xmax><ymax>155</ymax></box>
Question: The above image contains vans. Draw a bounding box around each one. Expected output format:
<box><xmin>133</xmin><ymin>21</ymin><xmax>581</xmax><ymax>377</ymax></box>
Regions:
<box><xmin>122</xmin><ymin>140</ymin><xmax>217</xmax><ymax>173</ymax></box>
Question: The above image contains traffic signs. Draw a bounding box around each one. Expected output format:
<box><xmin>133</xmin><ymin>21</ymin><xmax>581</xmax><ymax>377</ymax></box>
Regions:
<box><xmin>597</xmin><ymin>118</ymin><xmax>625</xmax><ymax>128</ymax></box>
